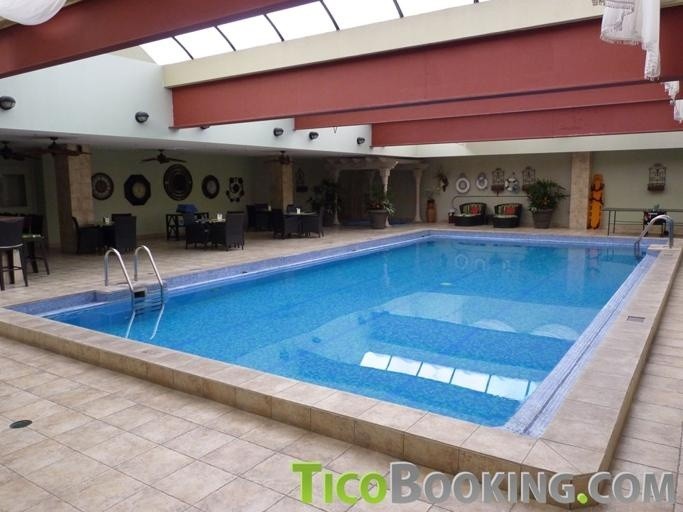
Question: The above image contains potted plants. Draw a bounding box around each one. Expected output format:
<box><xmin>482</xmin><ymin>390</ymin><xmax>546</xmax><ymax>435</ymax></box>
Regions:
<box><xmin>523</xmin><ymin>175</ymin><xmax>570</xmax><ymax>228</ymax></box>
<box><xmin>365</xmin><ymin>183</ymin><xmax>400</xmax><ymax>229</ymax></box>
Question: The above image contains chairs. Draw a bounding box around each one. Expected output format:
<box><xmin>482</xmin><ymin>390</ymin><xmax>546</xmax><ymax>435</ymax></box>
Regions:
<box><xmin>0</xmin><ymin>212</ymin><xmax>50</xmax><ymax>290</ymax></box>
<box><xmin>70</xmin><ymin>213</ymin><xmax>137</xmax><ymax>256</ymax></box>
<box><xmin>453</xmin><ymin>202</ymin><xmax>522</xmax><ymax>228</ymax></box>
<box><xmin>182</xmin><ymin>211</ymin><xmax>247</xmax><ymax>251</ymax></box>
<box><xmin>271</xmin><ymin>204</ymin><xmax>325</xmax><ymax>238</ymax></box>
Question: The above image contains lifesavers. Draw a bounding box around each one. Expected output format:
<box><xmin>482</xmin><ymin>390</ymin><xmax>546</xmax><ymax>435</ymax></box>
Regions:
<box><xmin>455</xmin><ymin>176</ymin><xmax>470</xmax><ymax>193</ymax></box>
<box><xmin>454</xmin><ymin>253</ymin><xmax>469</xmax><ymax>270</ymax></box>
<box><xmin>477</xmin><ymin>176</ymin><xmax>488</xmax><ymax>189</ymax></box>
<box><xmin>474</xmin><ymin>258</ymin><xmax>484</xmax><ymax>273</ymax></box>
<box><xmin>506</xmin><ymin>177</ymin><xmax>519</xmax><ymax>191</ymax></box>
<box><xmin>502</xmin><ymin>260</ymin><xmax>511</xmax><ymax>273</ymax></box>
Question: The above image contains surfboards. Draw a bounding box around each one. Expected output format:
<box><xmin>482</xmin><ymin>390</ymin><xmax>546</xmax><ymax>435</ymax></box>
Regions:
<box><xmin>590</xmin><ymin>174</ymin><xmax>604</xmax><ymax>228</ymax></box>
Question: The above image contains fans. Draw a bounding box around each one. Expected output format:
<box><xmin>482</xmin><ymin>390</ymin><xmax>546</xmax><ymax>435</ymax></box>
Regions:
<box><xmin>141</xmin><ymin>149</ymin><xmax>187</xmax><ymax>165</ymax></box>
<box><xmin>1</xmin><ymin>136</ymin><xmax>92</xmax><ymax>163</ymax></box>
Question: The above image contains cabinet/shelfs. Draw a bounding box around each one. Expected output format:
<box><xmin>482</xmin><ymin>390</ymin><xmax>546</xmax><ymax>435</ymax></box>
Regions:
<box><xmin>166</xmin><ymin>212</ymin><xmax>209</xmax><ymax>241</ymax></box>
